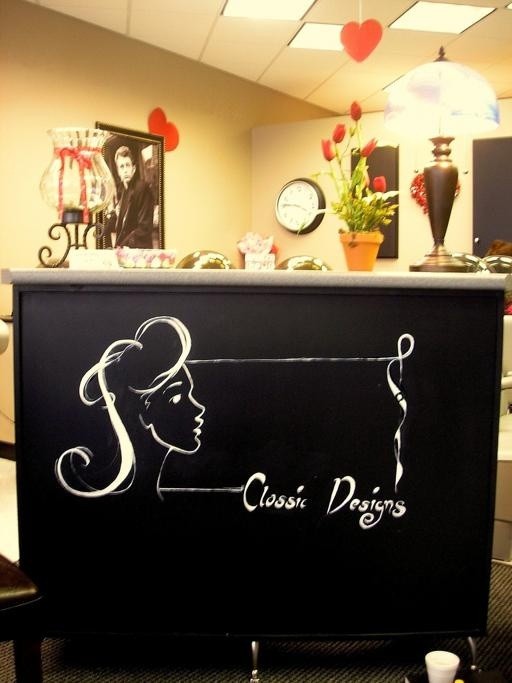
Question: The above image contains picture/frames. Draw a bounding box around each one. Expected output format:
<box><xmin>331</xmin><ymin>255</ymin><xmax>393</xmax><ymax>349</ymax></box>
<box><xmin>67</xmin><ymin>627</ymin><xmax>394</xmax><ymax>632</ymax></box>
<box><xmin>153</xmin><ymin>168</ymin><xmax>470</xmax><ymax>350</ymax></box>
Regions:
<box><xmin>96</xmin><ymin>121</ymin><xmax>165</xmax><ymax>250</ymax></box>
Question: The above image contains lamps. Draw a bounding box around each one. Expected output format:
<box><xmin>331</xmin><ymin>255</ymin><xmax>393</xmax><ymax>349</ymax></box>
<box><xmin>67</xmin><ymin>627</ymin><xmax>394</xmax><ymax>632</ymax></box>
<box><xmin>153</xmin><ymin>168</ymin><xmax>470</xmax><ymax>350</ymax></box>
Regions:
<box><xmin>385</xmin><ymin>45</ymin><xmax>501</xmax><ymax>271</ymax></box>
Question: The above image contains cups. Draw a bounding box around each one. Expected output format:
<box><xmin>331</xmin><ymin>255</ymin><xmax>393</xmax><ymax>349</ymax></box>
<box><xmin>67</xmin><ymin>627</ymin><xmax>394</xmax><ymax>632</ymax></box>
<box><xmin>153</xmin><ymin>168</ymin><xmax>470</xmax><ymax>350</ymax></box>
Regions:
<box><xmin>425</xmin><ymin>650</ymin><xmax>460</xmax><ymax>683</ymax></box>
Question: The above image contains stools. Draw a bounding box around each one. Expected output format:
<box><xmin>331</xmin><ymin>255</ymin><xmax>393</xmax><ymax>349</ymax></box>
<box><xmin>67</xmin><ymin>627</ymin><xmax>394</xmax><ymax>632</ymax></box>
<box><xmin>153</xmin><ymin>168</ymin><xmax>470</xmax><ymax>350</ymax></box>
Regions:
<box><xmin>0</xmin><ymin>557</ymin><xmax>43</xmax><ymax>683</ymax></box>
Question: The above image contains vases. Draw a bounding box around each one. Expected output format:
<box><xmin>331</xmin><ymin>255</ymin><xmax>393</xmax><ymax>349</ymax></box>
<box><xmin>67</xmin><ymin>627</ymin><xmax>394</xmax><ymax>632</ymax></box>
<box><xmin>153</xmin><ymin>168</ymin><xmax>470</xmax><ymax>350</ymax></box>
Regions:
<box><xmin>37</xmin><ymin>127</ymin><xmax>118</xmax><ymax>266</ymax></box>
<box><xmin>340</xmin><ymin>232</ymin><xmax>383</xmax><ymax>272</ymax></box>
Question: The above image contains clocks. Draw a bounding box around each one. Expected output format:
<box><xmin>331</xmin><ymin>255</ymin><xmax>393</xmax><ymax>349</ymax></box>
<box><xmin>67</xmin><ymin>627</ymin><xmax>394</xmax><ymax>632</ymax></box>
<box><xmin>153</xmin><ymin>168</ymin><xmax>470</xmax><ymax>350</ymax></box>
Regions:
<box><xmin>275</xmin><ymin>177</ymin><xmax>326</xmax><ymax>235</ymax></box>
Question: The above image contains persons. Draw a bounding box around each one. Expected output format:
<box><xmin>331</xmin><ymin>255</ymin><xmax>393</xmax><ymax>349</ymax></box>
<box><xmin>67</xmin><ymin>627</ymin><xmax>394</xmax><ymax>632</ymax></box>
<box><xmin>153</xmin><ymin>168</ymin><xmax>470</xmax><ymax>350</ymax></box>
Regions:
<box><xmin>485</xmin><ymin>238</ymin><xmax>512</xmax><ymax>315</ymax></box>
<box><xmin>102</xmin><ymin>143</ymin><xmax>155</xmax><ymax>249</ymax></box>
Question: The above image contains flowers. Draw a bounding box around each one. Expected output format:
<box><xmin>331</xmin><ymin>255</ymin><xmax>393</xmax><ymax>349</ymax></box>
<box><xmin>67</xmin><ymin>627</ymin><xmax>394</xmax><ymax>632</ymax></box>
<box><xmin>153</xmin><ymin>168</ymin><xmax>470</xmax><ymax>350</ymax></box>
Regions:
<box><xmin>315</xmin><ymin>102</ymin><xmax>400</xmax><ymax>232</ymax></box>
